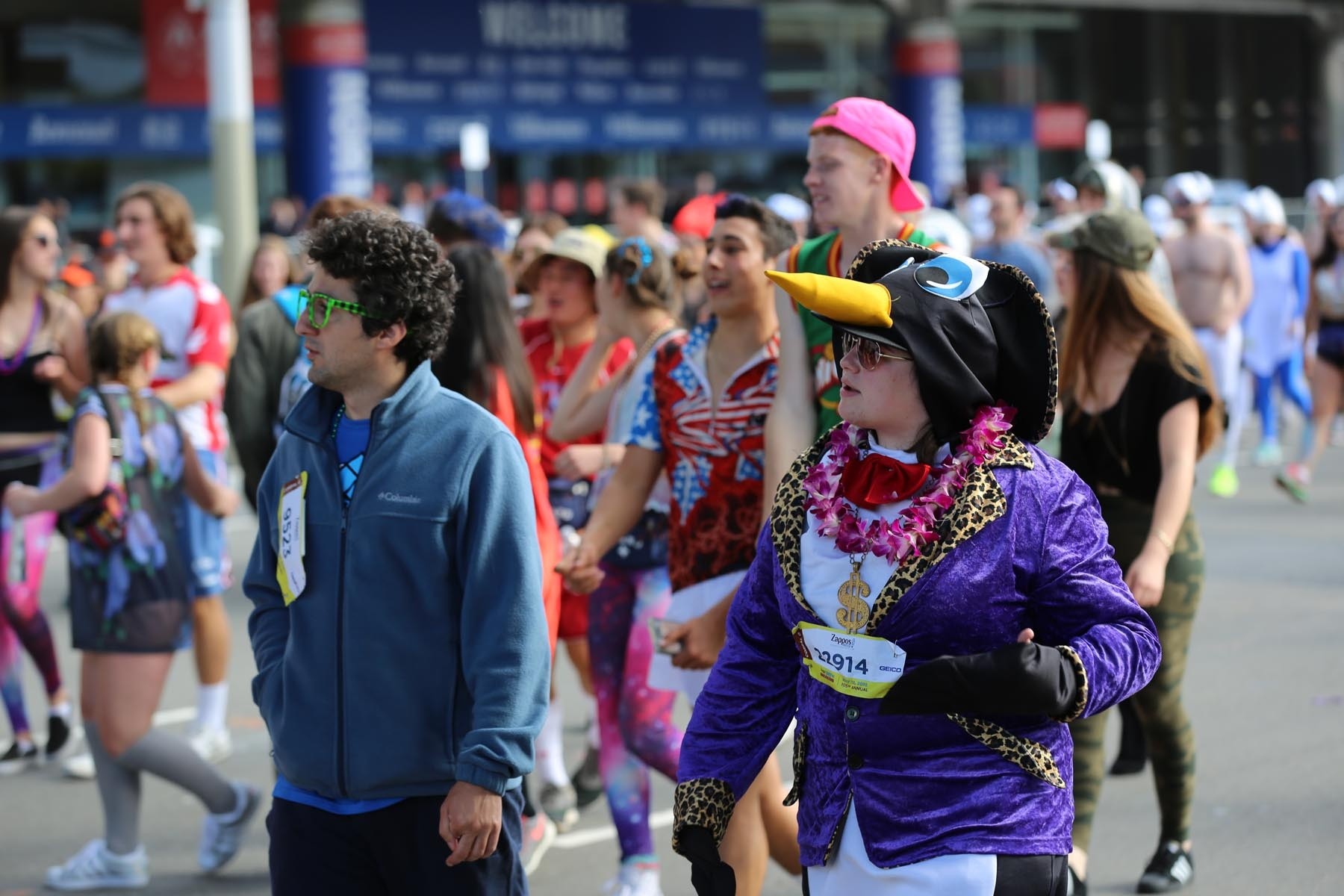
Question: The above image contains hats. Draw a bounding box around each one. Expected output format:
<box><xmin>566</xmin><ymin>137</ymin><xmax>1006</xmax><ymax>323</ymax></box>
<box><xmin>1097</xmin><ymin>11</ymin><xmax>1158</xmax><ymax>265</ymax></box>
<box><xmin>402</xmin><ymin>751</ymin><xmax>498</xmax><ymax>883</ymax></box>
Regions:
<box><xmin>804</xmin><ymin>96</ymin><xmax>923</xmax><ymax>212</ymax></box>
<box><xmin>1042</xmin><ymin>214</ymin><xmax>1156</xmax><ymax>268</ymax></box>
<box><xmin>508</xmin><ymin>226</ymin><xmax>609</xmax><ymax>296</ymax></box>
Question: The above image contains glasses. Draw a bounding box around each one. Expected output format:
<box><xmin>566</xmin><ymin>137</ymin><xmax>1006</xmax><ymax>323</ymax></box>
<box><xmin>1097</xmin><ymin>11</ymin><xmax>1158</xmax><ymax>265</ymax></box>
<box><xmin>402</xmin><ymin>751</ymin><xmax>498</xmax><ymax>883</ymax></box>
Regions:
<box><xmin>838</xmin><ymin>329</ymin><xmax>920</xmax><ymax>369</ymax></box>
<box><xmin>296</xmin><ymin>288</ymin><xmax>376</xmax><ymax>332</ymax></box>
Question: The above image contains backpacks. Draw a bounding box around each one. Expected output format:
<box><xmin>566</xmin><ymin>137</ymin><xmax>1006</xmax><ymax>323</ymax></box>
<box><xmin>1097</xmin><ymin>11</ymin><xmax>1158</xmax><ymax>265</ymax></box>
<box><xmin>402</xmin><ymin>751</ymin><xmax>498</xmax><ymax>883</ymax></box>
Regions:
<box><xmin>276</xmin><ymin>283</ymin><xmax>320</xmax><ymax>457</ymax></box>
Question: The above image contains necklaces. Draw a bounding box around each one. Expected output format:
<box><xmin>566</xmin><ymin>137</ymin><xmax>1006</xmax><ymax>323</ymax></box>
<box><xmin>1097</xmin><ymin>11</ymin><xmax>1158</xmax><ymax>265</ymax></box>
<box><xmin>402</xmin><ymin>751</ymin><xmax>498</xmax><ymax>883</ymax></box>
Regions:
<box><xmin>838</xmin><ymin>427</ymin><xmax>946</xmax><ymax>636</ymax></box>
<box><xmin>332</xmin><ymin>398</ymin><xmax>346</xmax><ymax>444</ymax></box>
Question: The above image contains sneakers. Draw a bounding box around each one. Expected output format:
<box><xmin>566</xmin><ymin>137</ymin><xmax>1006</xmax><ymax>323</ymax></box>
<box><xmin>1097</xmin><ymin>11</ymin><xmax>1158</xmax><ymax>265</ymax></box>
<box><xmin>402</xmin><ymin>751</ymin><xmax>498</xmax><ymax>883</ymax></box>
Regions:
<box><xmin>1136</xmin><ymin>845</ymin><xmax>1195</xmax><ymax>893</ymax></box>
<box><xmin>607</xmin><ymin>855</ymin><xmax>660</xmax><ymax>895</ymax></box>
<box><xmin>44</xmin><ymin>693</ymin><xmax>76</xmax><ymax>755</ymax></box>
<box><xmin>194</xmin><ymin>780</ymin><xmax>261</xmax><ymax>874</ymax></box>
<box><xmin>516</xmin><ymin>814</ymin><xmax>556</xmax><ymax>881</ymax></box>
<box><xmin>62</xmin><ymin>750</ymin><xmax>99</xmax><ymax>783</ymax></box>
<box><xmin>1280</xmin><ymin>465</ymin><xmax>1314</xmax><ymax>500</ymax></box>
<box><xmin>0</xmin><ymin>738</ymin><xmax>38</xmax><ymax>774</ymax></box>
<box><xmin>184</xmin><ymin>722</ymin><xmax>234</xmax><ymax>770</ymax></box>
<box><xmin>1208</xmin><ymin>466</ymin><xmax>1242</xmax><ymax>500</ymax></box>
<box><xmin>1068</xmin><ymin>864</ymin><xmax>1086</xmax><ymax>896</ymax></box>
<box><xmin>39</xmin><ymin>837</ymin><xmax>151</xmax><ymax>889</ymax></box>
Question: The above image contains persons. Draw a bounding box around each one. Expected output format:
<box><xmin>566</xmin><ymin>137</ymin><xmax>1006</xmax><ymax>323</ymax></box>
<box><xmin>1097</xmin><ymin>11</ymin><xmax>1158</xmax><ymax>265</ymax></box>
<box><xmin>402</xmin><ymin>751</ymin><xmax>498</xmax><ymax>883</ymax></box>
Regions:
<box><xmin>242</xmin><ymin>205</ymin><xmax>551</xmax><ymax>895</ymax></box>
<box><xmin>552</xmin><ymin>196</ymin><xmax>805</xmax><ymax>893</ymax></box>
<box><xmin>0</xmin><ymin>196</ymin><xmax>92</xmax><ymax>782</ymax></box>
<box><xmin>3</xmin><ymin>310</ymin><xmax>261</xmax><ymax>894</ymax></box>
<box><xmin>545</xmin><ymin>231</ymin><xmax>697</xmax><ymax>894</ymax></box>
<box><xmin>762</xmin><ymin>94</ymin><xmax>971</xmax><ymax>561</ymax></box>
<box><xmin>674</xmin><ymin>237</ymin><xmax>1164</xmax><ymax>895</ymax></box>
<box><xmin>428</xmin><ymin>244</ymin><xmax>558</xmax><ymax>883</ymax></box>
<box><xmin>0</xmin><ymin>130</ymin><xmax>1343</xmax><ymax>553</ymax></box>
<box><xmin>1039</xmin><ymin>211</ymin><xmax>1221</xmax><ymax>890</ymax></box>
<box><xmin>56</xmin><ymin>184</ymin><xmax>231</xmax><ymax>787</ymax></box>
<box><xmin>504</xmin><ymin>221</ymin><xmax>643</xmax><ymax>835</ymax></box>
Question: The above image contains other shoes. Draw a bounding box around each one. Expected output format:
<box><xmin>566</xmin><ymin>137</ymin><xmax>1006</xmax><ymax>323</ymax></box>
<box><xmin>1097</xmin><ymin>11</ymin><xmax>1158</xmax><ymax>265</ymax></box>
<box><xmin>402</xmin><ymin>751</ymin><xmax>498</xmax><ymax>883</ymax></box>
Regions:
<box><xmin>564</xmin><ymin>763</ymin><xmax>607</xmax><ymax>807</ymax></box>
